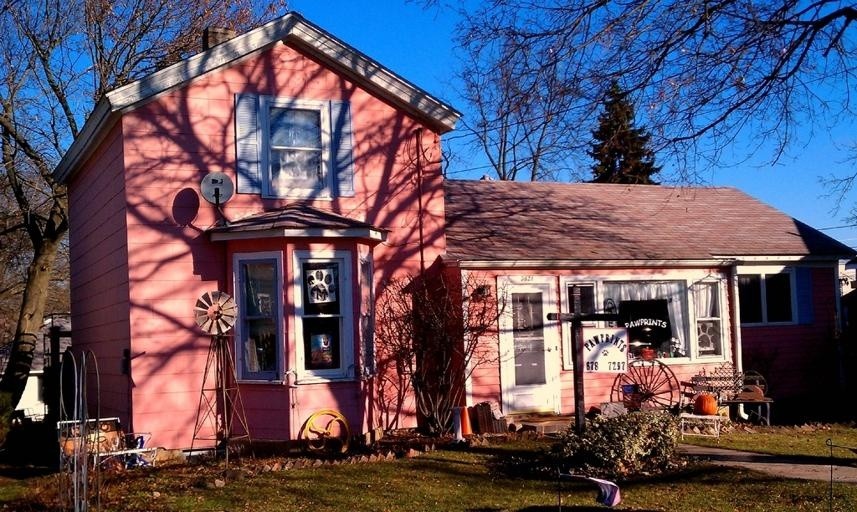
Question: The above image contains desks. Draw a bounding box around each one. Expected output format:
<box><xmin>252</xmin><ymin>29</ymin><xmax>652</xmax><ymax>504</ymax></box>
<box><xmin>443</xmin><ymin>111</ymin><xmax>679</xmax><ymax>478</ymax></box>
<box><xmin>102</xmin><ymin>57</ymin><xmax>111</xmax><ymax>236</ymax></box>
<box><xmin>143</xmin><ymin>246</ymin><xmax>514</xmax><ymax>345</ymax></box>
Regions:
<box><xmin>680</xmin><ymin>413</ymin><xmax>722</xmax><ymax>445</ymax></box>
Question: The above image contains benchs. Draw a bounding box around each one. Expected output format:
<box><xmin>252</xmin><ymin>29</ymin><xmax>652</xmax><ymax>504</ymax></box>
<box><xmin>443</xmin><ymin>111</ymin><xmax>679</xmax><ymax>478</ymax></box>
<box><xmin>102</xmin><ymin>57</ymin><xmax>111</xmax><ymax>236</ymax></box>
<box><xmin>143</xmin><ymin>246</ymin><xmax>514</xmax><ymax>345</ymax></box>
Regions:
<box><xmin>57</xmin><ymin>417</ymin><xmax>156</xmax><ymax>470</ymax></box>
<box><xmin>693</xmin><ymin>375</ymin><xmax>774</xmax><ymax>427</ymax></box>
